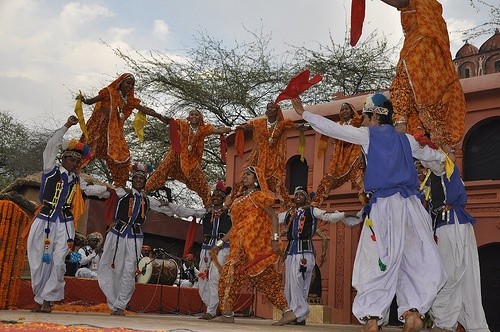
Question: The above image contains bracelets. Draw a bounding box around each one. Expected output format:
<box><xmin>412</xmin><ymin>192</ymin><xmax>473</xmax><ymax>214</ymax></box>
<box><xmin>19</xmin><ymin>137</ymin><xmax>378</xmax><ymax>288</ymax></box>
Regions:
<box><xmin>214</xmin><ymin>239</ymin><xmax>225</xmax><ymax>249</ymax></box>
<box><xmin>157</xmin><ymin>114</ymin><xmax>162</xmax><ymax>118</ymax></box>
<box><xmin>304</xmin><ymin>123</ymin><xmax>310</xmax><ymax>128</ymax></box>
<box><xmin>271</xmin><ymin>233</ymin><xmax>281</xmax><ymax>241</ymax></box>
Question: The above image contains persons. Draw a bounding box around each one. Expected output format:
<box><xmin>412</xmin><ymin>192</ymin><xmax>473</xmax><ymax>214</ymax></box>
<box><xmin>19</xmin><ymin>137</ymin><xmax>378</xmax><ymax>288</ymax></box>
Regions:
<box><xmin>221</xmin><ymin>101</ymin><xmax>310</xmax><ymax>210</ymax></box>
<box><xmin>143</xmin><ymin>106</ymin><xmax>245</xmax><ymax>213</ymax></box>
<box><xmin>174</xmin><ymin>253</ymin><xmax>205</xmax><ymax>288</ymax></box>
<box><xmin>21</xmin><ymin>115</ymin><xmax>114</xmax><ymax>314</ymax></box>
<box><xmin>73</xmin><ymin>73</ymin><xmax>167</xmax><ymax>187</ymax></box>
<box><xmin>208</xmin><ymin>166</ymin><xmax>296</xmax><ymax>326</ymax></box>
<box><xmin>406</xmin><ymin>102</ymin><xmax>426</xmax><ymax>137</ymax></box>
<box><xmin>381</xmin><ymin>0</ymin><xmax>467</xmax><ymax>147</ymax></box>
<box><xmin>291</xmin><ymin>92</ymin><xmax>456</xmax><ymax>331</ymax></box>
<box><xmin>277</xmin><ymin>186</ymin><xmax>359</xmax><ymax>325</ymax></box>
<box><xmin>413</xmin><ymin>132</ymin><xmax>491</xmax><ymax>332</ymax></box>
<box><xmin>86</xmin><ymin>162</ymin><xmax>176</xmax><ymax>316</ymax></box>
<box><xmin>323</xmin><ymin>204</ymin><xmax>390</xmax><ymax>330</ymax></box>
<box><xmin>139</xmin><ymin>245</ymin><xmax>153</xmax><ymax>262</ymax></box>
<box><xmin>158</xmin><ymin>177</ymin><xmax>233</xmax><ymax>321</ymax></box>
<box><xmin>65</xmin><ymin>231</ymin><xmax>104</xmax><ymax>278</ymax></box>
<box><xmin>294</xmin><ymin>102</ymin><xmax>370</xmax><ymax>209</ymax></box>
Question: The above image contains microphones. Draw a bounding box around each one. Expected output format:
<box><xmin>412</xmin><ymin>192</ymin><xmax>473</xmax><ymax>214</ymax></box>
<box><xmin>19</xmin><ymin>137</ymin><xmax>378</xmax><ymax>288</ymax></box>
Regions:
<box><xmin>153</xmin><ymin>248</ymin><xmax>164</xmax><ymax>252</ymax></box>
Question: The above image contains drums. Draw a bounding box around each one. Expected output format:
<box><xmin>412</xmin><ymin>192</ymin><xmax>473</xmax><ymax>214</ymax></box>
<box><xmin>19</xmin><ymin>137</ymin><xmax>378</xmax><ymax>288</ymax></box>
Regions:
<box><xmin>137</xmin><ymin>256</ymin><xmax>178</xmax><ymax>286</ymax></box>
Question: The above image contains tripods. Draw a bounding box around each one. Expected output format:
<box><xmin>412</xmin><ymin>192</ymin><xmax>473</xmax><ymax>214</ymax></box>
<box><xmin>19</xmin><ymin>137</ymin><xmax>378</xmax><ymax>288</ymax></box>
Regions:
<box><xmin>143</xmin><ymin>252</ymin><xmax>196</xmax><ymax>316</ymax></box>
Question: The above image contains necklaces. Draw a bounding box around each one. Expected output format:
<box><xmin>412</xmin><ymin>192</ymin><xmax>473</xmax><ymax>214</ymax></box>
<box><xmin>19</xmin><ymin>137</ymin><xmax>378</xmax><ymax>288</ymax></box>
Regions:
<box><xmin>226</xmin><ymin>187</ymin><xmax>259</xmax><ymax>216</ymax></box>
<box><xmin>187</xmin><ymin>120</ymin><xmax>200</xmax><ymax>152</ymax></box>
<box><xmin>266</xmin><ymin>117</ymin><xmax>278</xmax><ymax>143</ymax></box>
<box><xmin>117</xmin><ymin>89</ymin><xmax>130</xmax><ymax>119</ymax></box>
<box><xmin>343</xmin><ymin>118</ymin><xmax>353</xmax><ymax>126</ymax></box>
